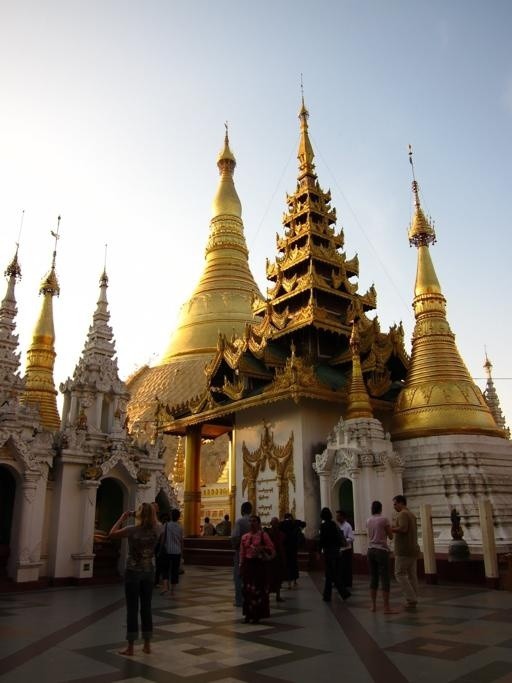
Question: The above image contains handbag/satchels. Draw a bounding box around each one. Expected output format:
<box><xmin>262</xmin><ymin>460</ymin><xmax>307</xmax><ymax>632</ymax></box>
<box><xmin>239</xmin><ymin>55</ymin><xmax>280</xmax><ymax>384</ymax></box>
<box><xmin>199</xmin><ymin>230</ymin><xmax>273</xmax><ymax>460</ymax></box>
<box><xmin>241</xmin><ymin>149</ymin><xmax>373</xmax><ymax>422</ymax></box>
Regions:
<box><xmin>153</xmin><ymin>524</ymin><xmax>168</xmax><ymax>561</ymax></box>
<box><xmin>256</xmin><ymin>531</ymin><xmax>274</xmax><ymax>564</ymax></box>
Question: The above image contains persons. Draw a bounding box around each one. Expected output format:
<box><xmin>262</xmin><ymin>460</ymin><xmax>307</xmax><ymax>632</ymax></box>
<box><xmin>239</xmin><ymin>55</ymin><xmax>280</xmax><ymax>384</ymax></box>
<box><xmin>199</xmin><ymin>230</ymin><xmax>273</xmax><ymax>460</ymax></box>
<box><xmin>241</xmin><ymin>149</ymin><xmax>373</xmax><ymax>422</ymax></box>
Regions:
<box><xmin>199</xmin><ymin>494</ymin><xmax>419</xmax><ymax>623</ymax></box>
<box><xmin>109</xmin><ymin>494</ymin><xmax>188</xmax><ymax>655</ymax></box>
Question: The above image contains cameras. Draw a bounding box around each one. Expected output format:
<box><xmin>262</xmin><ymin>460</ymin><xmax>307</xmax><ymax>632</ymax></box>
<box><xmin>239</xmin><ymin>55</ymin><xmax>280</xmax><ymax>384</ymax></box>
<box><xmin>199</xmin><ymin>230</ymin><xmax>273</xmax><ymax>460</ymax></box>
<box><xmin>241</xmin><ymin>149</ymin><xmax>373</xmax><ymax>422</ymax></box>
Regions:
<box><xmin>128</xmin><ymin>510</ymin><xmax>137</xmax><ymax>517</ymax></box>
